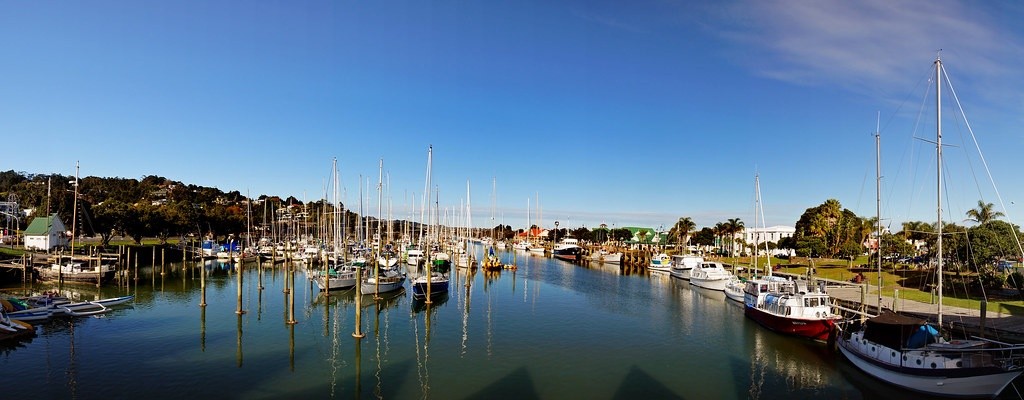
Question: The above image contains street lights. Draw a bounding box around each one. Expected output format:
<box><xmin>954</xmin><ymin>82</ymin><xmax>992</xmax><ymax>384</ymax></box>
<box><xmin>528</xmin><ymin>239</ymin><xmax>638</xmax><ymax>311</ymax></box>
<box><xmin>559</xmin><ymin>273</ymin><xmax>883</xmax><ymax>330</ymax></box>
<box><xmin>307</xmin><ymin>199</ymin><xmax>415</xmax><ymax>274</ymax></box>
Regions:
<box><xmin>68</xmin><ymin>160</ymin><xmax>81</xmax><ymax>264</ymax></box>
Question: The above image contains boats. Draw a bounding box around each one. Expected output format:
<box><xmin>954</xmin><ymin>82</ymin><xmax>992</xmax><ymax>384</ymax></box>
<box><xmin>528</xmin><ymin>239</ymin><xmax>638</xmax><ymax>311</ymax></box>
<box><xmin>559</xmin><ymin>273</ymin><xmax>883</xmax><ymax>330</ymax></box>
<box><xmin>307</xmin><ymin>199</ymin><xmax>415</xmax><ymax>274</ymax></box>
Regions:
<box><xmin>668</xmin><ymin>254</ymin><xmax>704</xmax><ymax>281</ymax></box>
<box><xmin>689</xmin><ymin>261</ymin><xmax>739</xmax><ymax>291</ymax></box>
<box><xmin>0</xmin><ymin>290</ymin><xmax>135</xmax><ymax>333</ymax></box>
<box><xmin>50</xmin><ymin>264</ymin><xmax>117</xmax><ymax>280</ymax></box>
<box><xmin>725</xmin><ymin>273</ymin><xmax>745</xmax><ymax>304</ymax></box>
<box><xmin>647</xmin><ymin>253</ymin><xmax>672</xmax><ymax>273</ymax></box>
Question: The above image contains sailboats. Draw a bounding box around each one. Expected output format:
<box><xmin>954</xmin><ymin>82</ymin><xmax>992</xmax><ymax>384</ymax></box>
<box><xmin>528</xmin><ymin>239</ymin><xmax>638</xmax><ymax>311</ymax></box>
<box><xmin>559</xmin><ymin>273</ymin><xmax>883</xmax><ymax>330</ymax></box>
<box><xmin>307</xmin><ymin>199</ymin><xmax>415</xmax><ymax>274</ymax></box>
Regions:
<box><xmin>200</xmin><ymin>144</ymin><xmax>623</xmax><ymax>296</ymax></box>
<box><xmin>743</xmin><ymin>172</ymin><xmax>842</xmax><ymax>343</ymax></box>
<box><xmin>834</xmin><ymin>45</ymin><xmax>1024</xmax><ymax>400</ymax></box>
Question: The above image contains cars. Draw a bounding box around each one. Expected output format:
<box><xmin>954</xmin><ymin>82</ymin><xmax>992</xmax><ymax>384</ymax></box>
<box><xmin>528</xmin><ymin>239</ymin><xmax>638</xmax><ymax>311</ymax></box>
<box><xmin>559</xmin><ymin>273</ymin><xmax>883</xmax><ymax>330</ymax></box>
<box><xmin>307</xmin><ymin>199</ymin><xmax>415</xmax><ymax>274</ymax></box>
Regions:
<box><xmin>997</xmin><ymin>260</ymin><xmax>1013</xmax><ymax>272</ymax></box>
<box><xmin>881</xmin><ymin>251</ymin><xmax>928</xmax><ymax>264</ymax></box>
